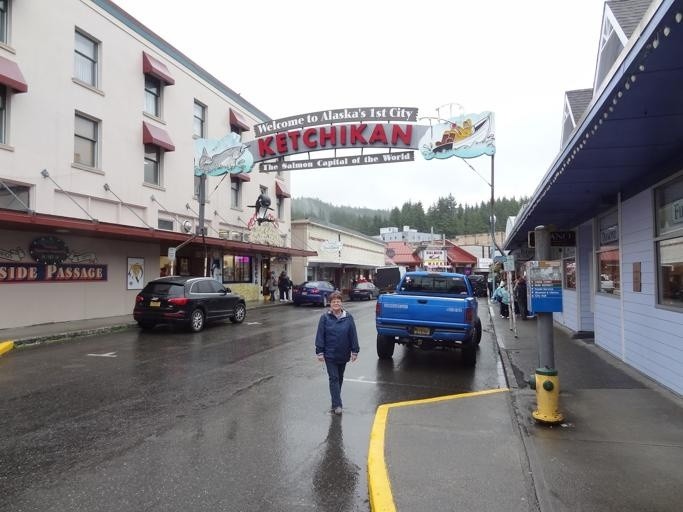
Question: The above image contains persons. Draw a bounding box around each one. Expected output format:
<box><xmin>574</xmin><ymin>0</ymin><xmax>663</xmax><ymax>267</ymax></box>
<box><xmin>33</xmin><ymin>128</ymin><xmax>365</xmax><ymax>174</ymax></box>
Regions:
<box><xmin>314</xmin><ymin>292</ymin><xmax>359</xmax><ymax>415</ymax></box>
<box><xmin>263</xmin><ymin>270</ymin><xmax>292</xmax><ymax>303</ymax></box>
<box><xmin>491</xmin><ymin>275</ymin><xmax>528</xmax><ymax>320</ymax></box>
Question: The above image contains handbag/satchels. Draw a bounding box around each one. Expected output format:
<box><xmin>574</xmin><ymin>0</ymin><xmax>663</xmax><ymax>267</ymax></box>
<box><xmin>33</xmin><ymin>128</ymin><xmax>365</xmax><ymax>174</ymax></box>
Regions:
<box><xmin>497</xmin><ymin>296</ymin><xmax>502</xmax><ymax>302</ymax></box>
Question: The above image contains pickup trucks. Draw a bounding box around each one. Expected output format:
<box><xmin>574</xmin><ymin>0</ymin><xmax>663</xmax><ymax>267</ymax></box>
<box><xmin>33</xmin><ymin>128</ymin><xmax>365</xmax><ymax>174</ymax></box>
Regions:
<box><xmin>375</xmin><ymin>271</ymin><xmax>482</xmax><ymax>366</ymax></box>
<box><xmin>601</xmin><ymin>274</ymin><xmax>615</xmax><ymax>294</ymax></box>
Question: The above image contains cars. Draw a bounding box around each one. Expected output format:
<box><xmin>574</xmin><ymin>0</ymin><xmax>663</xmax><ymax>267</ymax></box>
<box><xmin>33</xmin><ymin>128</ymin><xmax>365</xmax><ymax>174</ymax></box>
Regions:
<box><xmin>349</xmin><ymin>281</ymin><xmax>379</xmax><ymax>300</ymax></box>
<box><xmin>133</xmin><ymin>275</ymin><xmax>246</xmax><ymax>333</ymax></box>
<box><xmin>468</xmin><ymin>275</ymin><xmax>484</xmax><ymax>283</ymax></box>
<box><xmin>292</xmin><ymin>280</ymin><xmax>338</xmax><ymax>307</ymax></box>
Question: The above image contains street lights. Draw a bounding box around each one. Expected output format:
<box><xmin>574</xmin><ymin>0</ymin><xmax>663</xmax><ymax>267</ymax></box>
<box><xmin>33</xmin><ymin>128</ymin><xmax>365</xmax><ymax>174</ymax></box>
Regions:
<box><xmin>489</xmin><ymin>214</ymin><xmax>496</xmax><ymax>291</ymax></box>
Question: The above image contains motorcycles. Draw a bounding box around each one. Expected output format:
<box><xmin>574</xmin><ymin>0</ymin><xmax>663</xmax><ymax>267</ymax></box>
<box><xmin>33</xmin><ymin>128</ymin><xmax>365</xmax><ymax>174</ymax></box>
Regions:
<box><xmin>474</xmin><ymin>287</ymin><xmax>488</xmax><ymax>296</ymax></box>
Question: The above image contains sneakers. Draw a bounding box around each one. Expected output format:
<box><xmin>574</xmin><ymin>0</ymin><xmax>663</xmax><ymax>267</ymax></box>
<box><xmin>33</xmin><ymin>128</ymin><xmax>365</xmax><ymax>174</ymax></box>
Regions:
<box><xmin>499</xmin><ymin>315</ymin><xmax>509</xmax><ymax>319</ymax></box>
<box><xmin>334</xmin><ymin>406</ymin><xmax>342</xmax><ymax>414</ymax></box>
